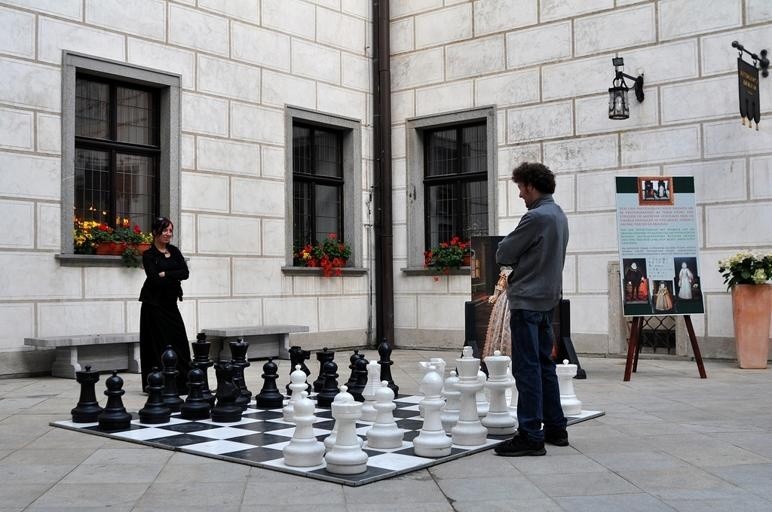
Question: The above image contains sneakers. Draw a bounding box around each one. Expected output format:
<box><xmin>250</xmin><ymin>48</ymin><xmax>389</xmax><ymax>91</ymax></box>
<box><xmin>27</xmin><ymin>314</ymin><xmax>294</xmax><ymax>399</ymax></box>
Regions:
<box><xmin>494</xmin><ymin>427</ymin><xmax>569</xmax><ymax>457</ymax></box>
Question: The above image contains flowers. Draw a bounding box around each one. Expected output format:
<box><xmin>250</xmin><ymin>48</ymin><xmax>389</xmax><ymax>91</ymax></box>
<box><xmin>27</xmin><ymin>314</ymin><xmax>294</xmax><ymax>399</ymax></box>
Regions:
<box><xmin>72</xmin><ymin>216</ymin><xmax>154</xmax><ymax>267</ymax></box>
<box><xmin>423</xmin><ymin>235</ymin><xmax>470</xmax><ymax>282</ymax></box>
<box><xmin>293</xmin><ymin>233</ymin><xmax>353</xmax><ymax>278</ymax></box>
<box><xmin>714</xmin><ymin>251</ymin><xmax>772</xmax><ymax>293</ymax></box>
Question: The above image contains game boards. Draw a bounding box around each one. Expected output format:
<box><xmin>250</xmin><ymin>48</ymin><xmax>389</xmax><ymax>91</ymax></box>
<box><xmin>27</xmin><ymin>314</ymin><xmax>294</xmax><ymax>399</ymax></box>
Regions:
<box><xmin>49</xmin><ymin>387</ymin><xmax>605</xmax><ymax>488</ymax></box>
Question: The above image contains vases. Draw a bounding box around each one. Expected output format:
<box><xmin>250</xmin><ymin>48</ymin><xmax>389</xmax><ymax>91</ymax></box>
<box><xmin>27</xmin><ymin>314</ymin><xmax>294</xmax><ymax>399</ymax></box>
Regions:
<box><xmin>731</xmin><ymin>284</ymin><xmax>772</xmax><ymax>369</ymax></box>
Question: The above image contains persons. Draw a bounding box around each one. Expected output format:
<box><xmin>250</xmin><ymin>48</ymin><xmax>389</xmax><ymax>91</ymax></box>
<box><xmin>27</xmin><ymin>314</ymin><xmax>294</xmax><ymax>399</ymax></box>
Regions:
<box><xmin>678</xmin><ymin>262</ymin><xmax>693</xmax><ymax>300</ymax></box>
<box><xmin>627</xmin><ymin>262</ymin><xmax>642</xmax><ymax>301</ymax></box>
<box><xmin>656</xmin><ymin>281</ymin><xmax>673</xmax><ymax>311</ymax></box>
<box><xmin>139</xmin><ymin>217</ymin><xmax>191</xmax><ymax>395</ymax></box>
<box><xmin>481</xmin><ymin>162</ymin><xmax>569</xmax><ymax>457</ymax></box>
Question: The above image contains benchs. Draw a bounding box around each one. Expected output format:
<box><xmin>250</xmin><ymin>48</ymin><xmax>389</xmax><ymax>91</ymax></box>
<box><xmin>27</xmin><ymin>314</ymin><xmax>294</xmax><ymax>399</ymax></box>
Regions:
<box><xmin>201</xmin><ymin>322</ymin><xmax>310</xmax><ymax>365</ymax></box>
<box><xmin>23</xmin><ymin>331</ymin><xmax>145</xmax><ymax>380</ymax></box>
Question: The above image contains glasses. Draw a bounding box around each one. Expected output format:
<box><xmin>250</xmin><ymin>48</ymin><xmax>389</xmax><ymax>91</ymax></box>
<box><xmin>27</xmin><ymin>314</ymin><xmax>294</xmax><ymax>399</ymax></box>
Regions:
<box><xmin>156</xmin><ymin>216</ymin><xmax>165</xmax><ymax>221</ymax></box>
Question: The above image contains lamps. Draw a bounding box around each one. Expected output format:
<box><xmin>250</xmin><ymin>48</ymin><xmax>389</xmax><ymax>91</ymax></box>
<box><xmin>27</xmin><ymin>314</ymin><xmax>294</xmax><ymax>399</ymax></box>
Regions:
<box><xmin>606</xmin><ymin>57</ymin><xmax>645</xmax><ymax>119</ymax></box>
